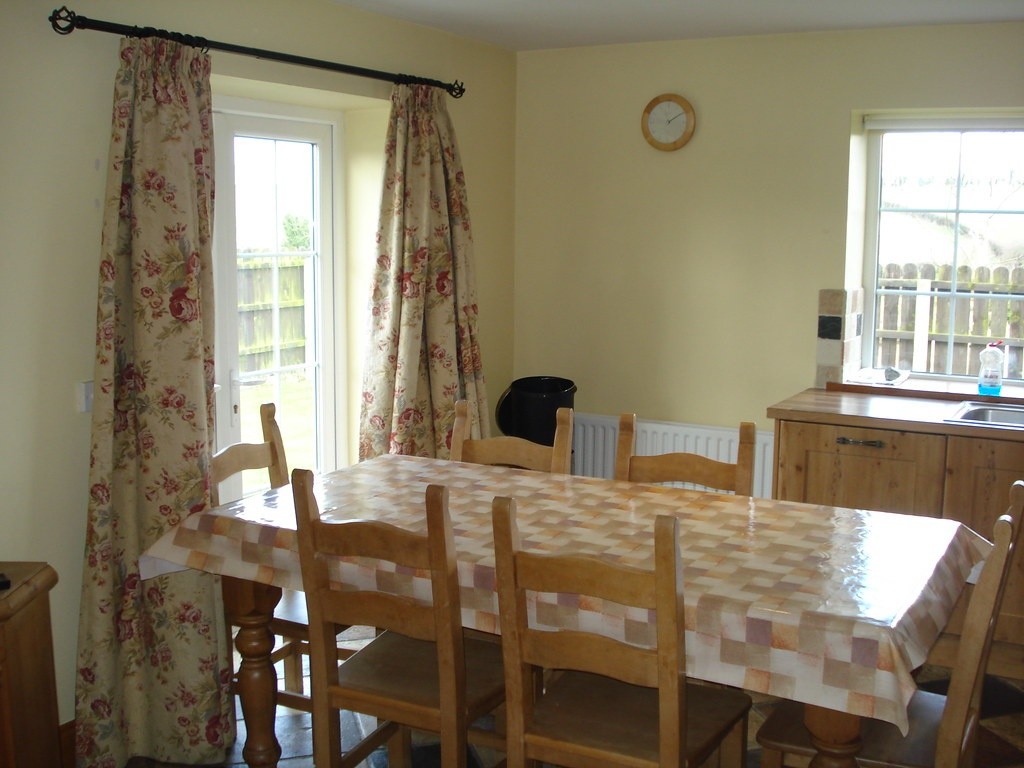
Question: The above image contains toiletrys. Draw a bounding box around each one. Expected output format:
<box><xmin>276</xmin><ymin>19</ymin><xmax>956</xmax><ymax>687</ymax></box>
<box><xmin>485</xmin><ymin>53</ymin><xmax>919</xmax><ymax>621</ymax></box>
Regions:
<box><xmin>976</xmin><ymin>340</ymin><xmax>1005</xmax><ymax>397</ymax></box>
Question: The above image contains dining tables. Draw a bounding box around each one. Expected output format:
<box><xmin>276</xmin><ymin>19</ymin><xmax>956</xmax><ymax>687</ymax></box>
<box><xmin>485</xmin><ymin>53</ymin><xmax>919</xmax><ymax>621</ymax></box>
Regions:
<box><xmin>139</xmin><ymin>453</ymin><xmax>995</xmax><ymax>768</ymax></box>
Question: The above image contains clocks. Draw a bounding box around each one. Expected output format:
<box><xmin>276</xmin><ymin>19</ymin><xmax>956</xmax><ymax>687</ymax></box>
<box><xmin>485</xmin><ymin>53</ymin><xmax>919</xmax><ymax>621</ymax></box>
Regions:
<box><xmin>641</xmin><ymin>93</ymin><xmax>695</xmax><ymax>152</ymax></box>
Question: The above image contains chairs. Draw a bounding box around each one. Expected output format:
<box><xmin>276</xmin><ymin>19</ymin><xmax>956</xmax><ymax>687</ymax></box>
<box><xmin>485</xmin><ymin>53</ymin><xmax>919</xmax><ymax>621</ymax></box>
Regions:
<box><xmin>449</xmin><ymin>399</ymin><xmax>574</xmax><ymax>475</ymax></box>
<box><xmin>614</xmin><ymin>413</ymin><xmax>756</xmax><ymax>497</ymax></box>
<box><xmin>291</xmin><ymin>469</ymin><xmax>541</xmax><ymax>768</ymax></box>
<box><xmin>210</xmin><ymin>403</ymin><xmax>387</xmax><ymax>742</ymax></box>
<box><xmin>756</xmin><ymin>480</ymin><xmax>1024</xmax><ymax>768</ymax></box>
<box><xmin>492</xmin><ymin>496</ymin><xmax>753</xmax><ymax>768</ymax></box>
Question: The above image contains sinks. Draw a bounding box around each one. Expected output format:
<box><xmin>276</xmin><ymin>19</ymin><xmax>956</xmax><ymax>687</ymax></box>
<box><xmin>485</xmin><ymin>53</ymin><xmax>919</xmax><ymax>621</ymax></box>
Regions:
<box><xmin>943</xmin><ymin>400</ymin><xmax>1024</xmax><ymax>431</ymax></box>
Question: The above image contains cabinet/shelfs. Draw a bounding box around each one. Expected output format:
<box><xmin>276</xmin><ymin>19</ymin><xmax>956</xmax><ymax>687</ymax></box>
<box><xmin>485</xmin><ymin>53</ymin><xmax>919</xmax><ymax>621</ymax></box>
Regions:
<box><xmin>766</xmin><ymin>387</ymin><xmax>1024</xmax><ymax>645</ymax></box>
<box><xmin>0</xmin><ymin>560</ymin><xmax>64</xmax><ymax>768</ymax></box>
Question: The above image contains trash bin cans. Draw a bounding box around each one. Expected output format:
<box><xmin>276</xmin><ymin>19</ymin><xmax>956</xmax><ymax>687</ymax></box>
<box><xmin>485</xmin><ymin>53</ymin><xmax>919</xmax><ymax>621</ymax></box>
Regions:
<box><xmin>495</xmin><ymin>375</ymin><xmax>578</xmax><ymax>454</ymax></box>
